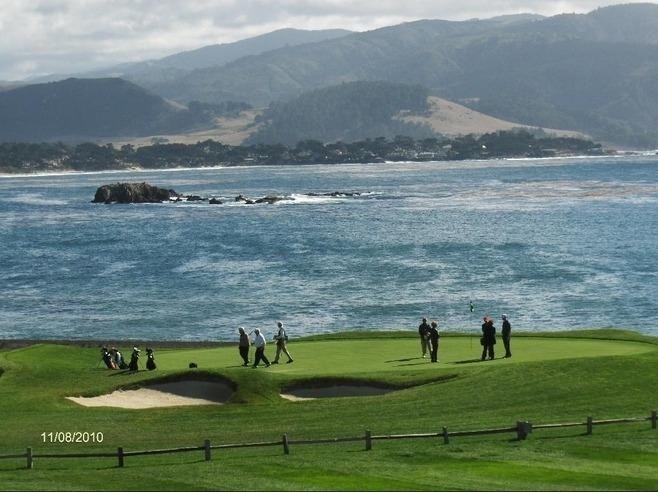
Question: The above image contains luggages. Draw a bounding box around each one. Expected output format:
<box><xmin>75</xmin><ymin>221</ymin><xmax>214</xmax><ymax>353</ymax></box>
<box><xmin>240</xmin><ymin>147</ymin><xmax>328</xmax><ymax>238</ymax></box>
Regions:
<box><xmin>96</xmin><ymin>346</ymin><xmax>156</xmax><ymax>371</ymax></box>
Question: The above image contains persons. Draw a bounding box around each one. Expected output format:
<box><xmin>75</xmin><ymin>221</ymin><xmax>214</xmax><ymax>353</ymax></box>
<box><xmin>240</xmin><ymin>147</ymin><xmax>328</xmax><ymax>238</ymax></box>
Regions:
<box><xmin>419</xmin><ymin>316</ymin><xmax>432</xmax><ymax>356</ymax></box>
<box><xmin>100</xmin><ymin>345</ymin><xmax>158</xmax><ymax>370</ymax></box>
<box><xmin>482</xmin><ymin>320</ymin><xmax>497</xmax><ymax>359</ymax></box>
<box><xmin>430</xmin><ymin>320</ymin><xmax>440</xmax><ymax>363</ymax></box>
<box><xmin>500</xmin><ymin>313</ymin><xmax>513</xmax><ymax>359</ymax></box>
<box><xmin>482</xmin><ymin>317</ymin><xmax>491</xmax><ymax>359</ymax></box>
<box><xmin>251</xmin><ymin>328</ymin><xmax>271</xmax><ymax>368</ymax></box>
<box><xmin>238</xmin><ymin>328</ymin><xmax>250</xmax><ymax>366</ymax></box>
<box><xmin>271</xmin><ymin>321</ymin><xmax>294</xmax><ymax>365</ymax></box>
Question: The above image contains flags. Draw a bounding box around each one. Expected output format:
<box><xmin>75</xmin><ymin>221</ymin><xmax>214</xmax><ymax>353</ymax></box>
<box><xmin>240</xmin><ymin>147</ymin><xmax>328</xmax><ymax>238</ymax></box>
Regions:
<box><xmin>470</xmin><ymin>300</ymin><xmax>474</xmax><ymax>312</ymax></box>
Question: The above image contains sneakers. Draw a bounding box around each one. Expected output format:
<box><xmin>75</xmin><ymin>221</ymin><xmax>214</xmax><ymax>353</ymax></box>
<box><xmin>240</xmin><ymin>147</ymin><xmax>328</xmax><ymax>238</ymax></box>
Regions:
<box><xmin>482</xmin><ymin>355</ymin><xmax>511</xmax><ymax>360</ymax></box>
<box><xmin>421</xmin><ymin>354</ymin><xmax>440</xmax><ymax>363</ymax></box>
<box><xmin>242</xmin><ymin>360</ymin><xmax>293</xmax><ymax>368</ymax></box>
